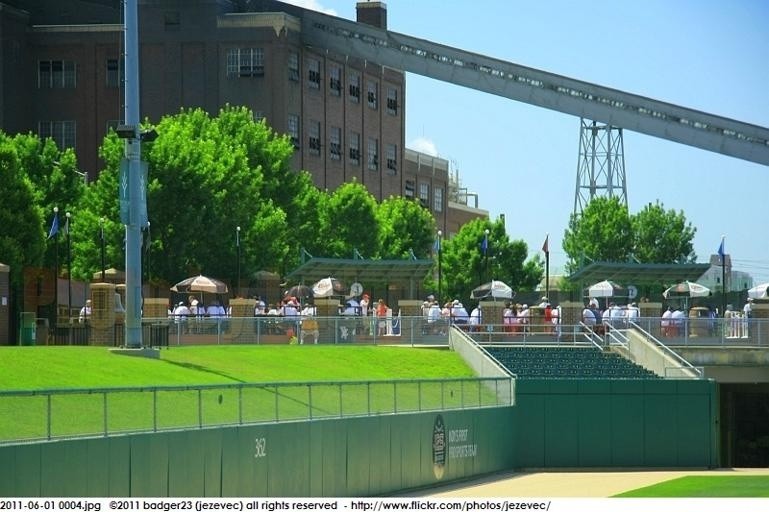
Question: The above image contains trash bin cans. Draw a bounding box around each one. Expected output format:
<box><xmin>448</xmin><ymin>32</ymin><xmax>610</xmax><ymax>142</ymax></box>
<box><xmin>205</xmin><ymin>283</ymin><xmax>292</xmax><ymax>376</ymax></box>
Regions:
<box><xmin>149</xmin><ymin>322</ymin><xmax>169</xmax><ymax>349</ymax></box>
<box><xmin>19</xmin><ymin>312</ymin><xmax>49</xmax><ymax>345</ymax></box>
<box><xmin>687</xmin><ymin>306</ymin><xmax>711</xmax><ymax>336</ymax></box>
<box><xmin>338</xmin><ymin>314</ymin><xmax>357</xmax><ymax>344</ymax></box>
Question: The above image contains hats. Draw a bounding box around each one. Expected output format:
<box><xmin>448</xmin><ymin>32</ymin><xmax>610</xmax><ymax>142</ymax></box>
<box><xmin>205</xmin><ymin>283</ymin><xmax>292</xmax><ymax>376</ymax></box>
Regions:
<box><xmin>541</xmin><ymin>296</ymin><xmax>548</xmax><ymax>301</ymax></box>
<box><xmin>427</xmin><ymin>294</ymin><xmax>435</xmax><ymax>299</ymax></box>
<box><xmin>363</xmin><ymin>293</ymin><xmax>369</xmax><ymax>298</ymax></box>
<box><xmin>747</xmin><ymin>297</ymin><xmax>754</xmax><ymax>301</ymax></box>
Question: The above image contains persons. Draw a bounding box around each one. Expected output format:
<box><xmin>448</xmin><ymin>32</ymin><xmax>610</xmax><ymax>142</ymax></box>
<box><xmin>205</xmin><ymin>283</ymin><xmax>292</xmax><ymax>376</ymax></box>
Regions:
<box><xmin>340</xmin><ymin>293</ymin><xmax>388</xmax><ymax>335</ymax></box>
<box><xmin>168</xmin><ymin>294</ymin><xmax>225</xmax><ymax>329</ymax></box>
<box><xmin>582</xmin><ymin>296</ymin><xmax>638</xmax><ymax>328</ymax></box>
<box><xmin>424</xmin><ymin>293</ymin><xmax>471</xmax><ymax>334</ymax></box>
<box><xmin>660</xmin><ymin>304</ymin><xmax>691</xmax><ymax>337</ymax></box>
<box><xmin>504</xmin><ymin>298</ymin><xmax>553</xmax><ymax>333</ymax></box>
<box><xmin>78</xmin><ymin>298</ymin><xmax>92</xmax><ymax>324</ymax></box>
<box><xmin>252</xmin><ymin>295</ymin><xmax>318</xmax><ymax>317</ymax></box>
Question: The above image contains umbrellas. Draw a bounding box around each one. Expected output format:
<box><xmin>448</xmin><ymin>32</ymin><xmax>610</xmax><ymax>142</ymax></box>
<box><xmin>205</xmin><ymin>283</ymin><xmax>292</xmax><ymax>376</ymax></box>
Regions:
<box><xmin>471</xmin><ymin>278</ymin><xmax>517</xmax><ymax>301</ymax></box>
<box><xmin>167</xmin><ymin>273</ymin><xmax>230</xmax><ymax>307</ymax></box>
<box><xmin>666</xmin><ymin>280</ymin><xmax>713</xmax><ymax>314</ymax></box>
<box><xmin>582</xmin><ymin>278</ymin><xmax>630</xmax><ymax>309</ymax></box>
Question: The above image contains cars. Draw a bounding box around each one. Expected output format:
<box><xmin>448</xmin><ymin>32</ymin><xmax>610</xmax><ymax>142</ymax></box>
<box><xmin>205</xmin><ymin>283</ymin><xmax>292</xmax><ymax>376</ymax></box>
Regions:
<box><xmin>392</xmin><ymin>319</ymin><xmax>399</xmax><ymax>334</ymax></box>
